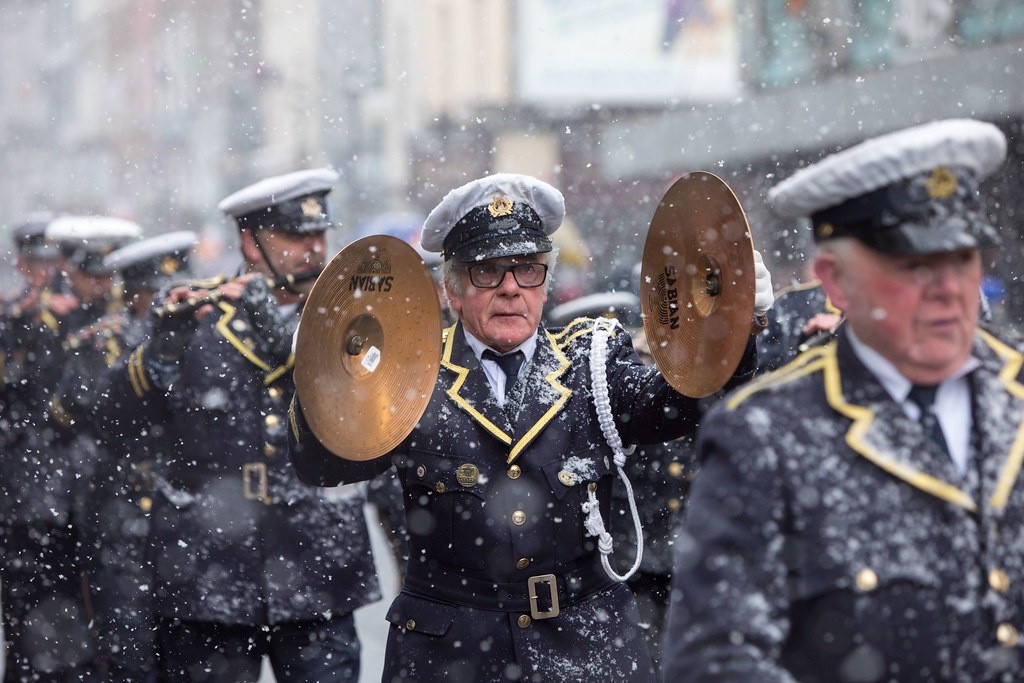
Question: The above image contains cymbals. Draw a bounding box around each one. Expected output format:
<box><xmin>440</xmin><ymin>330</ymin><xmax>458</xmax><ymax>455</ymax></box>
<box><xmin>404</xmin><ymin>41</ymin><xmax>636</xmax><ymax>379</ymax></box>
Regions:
<box><xmin>638</xmin><ymin>170</ymin><xmax>768</xmax><ymax>400</ymax></box>
<box><xmin>293</xmin><ymin>233</ymin><xmax>443</xmax><ymax>462</ymax></box>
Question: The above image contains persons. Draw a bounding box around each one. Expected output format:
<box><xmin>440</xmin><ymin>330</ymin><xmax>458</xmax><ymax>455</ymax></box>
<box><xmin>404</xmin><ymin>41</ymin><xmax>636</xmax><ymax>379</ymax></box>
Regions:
<box><xmin>1</xmin><ymin>117</ymin><xmax>1024</xmax><ymax>683</ymax></box>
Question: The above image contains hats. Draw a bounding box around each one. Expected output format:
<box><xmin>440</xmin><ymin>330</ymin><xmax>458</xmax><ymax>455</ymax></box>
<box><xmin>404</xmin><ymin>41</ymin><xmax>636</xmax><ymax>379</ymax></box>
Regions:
<box><xmin>420</xmin><ymin>174</ymin><xmax>566</xmax><ymax>263</ymax></box>
<box><xmin>220</xmin><ymin>169</ymin><xmax>343</xmax><ymax>230</ymax></box>
<box><xmin>12</xmin><ymin>221</ymin><xmax>47</xmax><ymax>259</ymax></box>
<box><xmin>551</xmin><ymin>291</ymin><xmax>642</xmax><ymax>327</ymax></box>
<box><xmin>105</xmin><ymin>231</ymin><xmax>197</xmax><ymax>289</ymax></box>
<box><xmin>769</xmin><ymin>119</ymin><xmax>1007</xmax><ymax>255</ymax></box>
<box><xmin>47</xmin><ymin>218</ymin><xmax>142</xmax><ymax>278</ymax></box>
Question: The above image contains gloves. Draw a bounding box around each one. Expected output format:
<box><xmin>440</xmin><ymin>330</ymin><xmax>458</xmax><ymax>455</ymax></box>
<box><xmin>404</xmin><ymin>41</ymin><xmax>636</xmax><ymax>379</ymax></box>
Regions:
<box><xmin>754</xmin><ymin>252</ymin><xmax>775</xmax><ymax>318</ymax></box>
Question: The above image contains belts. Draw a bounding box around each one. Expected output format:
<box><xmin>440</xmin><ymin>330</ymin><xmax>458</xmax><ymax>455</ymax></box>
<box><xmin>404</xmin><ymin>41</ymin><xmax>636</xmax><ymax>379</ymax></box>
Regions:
<box><xmin>237</xmin><ymin>464</ymin><xmax>300</xmax><ymax>503</ymax></box>
<box><xmin>412</xmin><ymin>571</ymin><xmax>600</xmax><ymax>621</ymax></box>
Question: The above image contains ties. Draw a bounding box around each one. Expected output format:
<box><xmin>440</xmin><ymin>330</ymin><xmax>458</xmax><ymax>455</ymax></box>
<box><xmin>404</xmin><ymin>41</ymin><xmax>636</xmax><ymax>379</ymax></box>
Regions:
<box><xmin>910</xmin><ymin>384</ymin><xmax>953</xmax><ymax>464</ymax></box>
<box><xmin>484</xmin><ymin>350</ymin><xmax>523</xmax><ymax>421</ymax></box>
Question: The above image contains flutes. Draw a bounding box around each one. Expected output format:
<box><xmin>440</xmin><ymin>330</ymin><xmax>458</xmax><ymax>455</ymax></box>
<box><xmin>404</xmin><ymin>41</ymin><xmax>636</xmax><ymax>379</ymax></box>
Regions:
<box><xmin>150</xmin><ymin>265</ymin><xmax>325</xmax><ymax>320</ymax></box>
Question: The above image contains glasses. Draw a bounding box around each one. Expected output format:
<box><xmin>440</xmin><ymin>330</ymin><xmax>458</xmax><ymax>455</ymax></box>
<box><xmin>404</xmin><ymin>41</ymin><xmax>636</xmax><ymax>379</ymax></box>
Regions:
<box><xmin>465</xmin><ymin>262</ymin><xmax>549</xmax><ymax>288</ymax></box>
